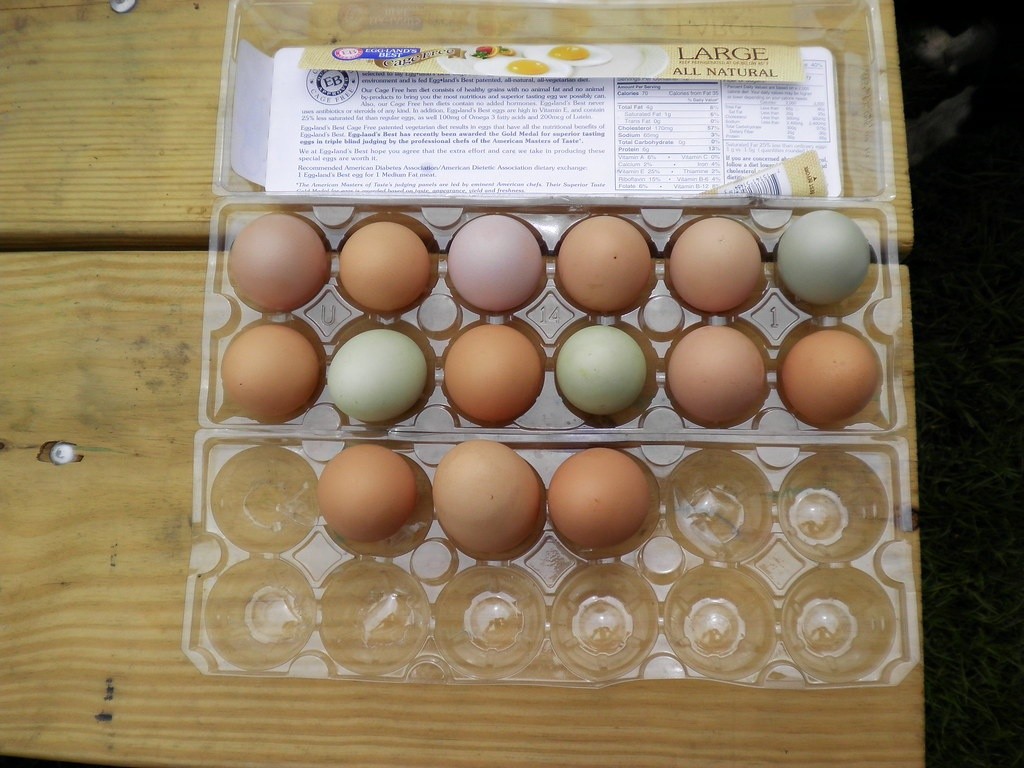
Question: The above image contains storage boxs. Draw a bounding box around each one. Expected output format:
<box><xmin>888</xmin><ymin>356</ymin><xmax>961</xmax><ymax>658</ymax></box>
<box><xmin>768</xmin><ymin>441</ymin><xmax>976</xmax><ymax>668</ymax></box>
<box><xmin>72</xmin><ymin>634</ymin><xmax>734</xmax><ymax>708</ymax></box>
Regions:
<box><xmin>178</xmin><ymin>0</ymin><xmax>922</xmax><ymax>692</ymax></box>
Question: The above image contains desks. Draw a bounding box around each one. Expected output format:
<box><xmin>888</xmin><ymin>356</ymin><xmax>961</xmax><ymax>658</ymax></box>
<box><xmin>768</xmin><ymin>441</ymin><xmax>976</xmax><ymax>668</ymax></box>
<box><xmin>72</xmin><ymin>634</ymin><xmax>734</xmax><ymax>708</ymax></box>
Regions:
<box><xmin>1</xmin><ymin>0</ymin><xmax>929</xmax><ymax>768</ymax></box>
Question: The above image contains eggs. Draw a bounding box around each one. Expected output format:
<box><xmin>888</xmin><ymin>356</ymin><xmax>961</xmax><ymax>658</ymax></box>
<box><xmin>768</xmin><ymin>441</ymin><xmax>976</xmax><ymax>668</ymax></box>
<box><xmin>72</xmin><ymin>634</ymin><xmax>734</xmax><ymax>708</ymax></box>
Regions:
<box><xmin>223</xmin><ymin>206</ymin><xmax>889</xmax><ymax>551</ymax></box>
